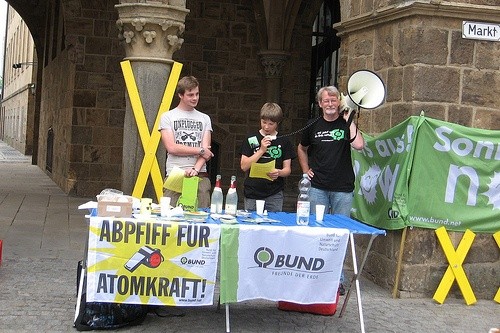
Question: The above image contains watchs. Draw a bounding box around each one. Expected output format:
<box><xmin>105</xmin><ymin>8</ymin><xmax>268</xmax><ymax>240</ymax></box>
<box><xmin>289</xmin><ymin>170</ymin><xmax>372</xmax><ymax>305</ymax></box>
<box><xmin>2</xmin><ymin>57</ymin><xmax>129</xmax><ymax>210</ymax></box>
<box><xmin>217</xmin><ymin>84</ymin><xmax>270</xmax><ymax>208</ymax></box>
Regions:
<box><xmin>198</xmin><ymin>146</ymin><xmax>206</xmax><ymax>155</ymax></box>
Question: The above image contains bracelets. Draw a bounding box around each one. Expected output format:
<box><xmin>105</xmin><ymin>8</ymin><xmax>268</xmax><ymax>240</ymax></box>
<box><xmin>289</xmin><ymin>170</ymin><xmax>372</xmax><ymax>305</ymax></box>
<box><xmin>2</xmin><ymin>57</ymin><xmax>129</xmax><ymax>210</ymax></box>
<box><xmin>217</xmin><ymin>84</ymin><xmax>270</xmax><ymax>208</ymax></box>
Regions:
<box><xmin>191</xmin><ymin>167</ymin><xmax>199</xmax><ymax>175</ymax></box>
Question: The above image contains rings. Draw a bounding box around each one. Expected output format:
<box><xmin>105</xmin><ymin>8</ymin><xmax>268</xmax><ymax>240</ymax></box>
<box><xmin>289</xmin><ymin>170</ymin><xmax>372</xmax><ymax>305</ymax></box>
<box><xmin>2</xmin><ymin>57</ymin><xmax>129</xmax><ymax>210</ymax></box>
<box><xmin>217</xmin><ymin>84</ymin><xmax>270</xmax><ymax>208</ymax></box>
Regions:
<box><xmin>208</xmin><ymin>159</ymin><xmax>210</xmax><ymax>160</ymax></box>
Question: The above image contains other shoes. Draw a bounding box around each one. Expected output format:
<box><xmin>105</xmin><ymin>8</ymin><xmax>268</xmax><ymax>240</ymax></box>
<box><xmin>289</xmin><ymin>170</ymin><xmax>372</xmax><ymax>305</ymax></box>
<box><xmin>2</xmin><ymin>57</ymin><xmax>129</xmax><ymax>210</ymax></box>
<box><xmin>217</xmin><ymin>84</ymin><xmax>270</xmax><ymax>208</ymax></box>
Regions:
<box><xmin>339</xmin><ymin>283</ymin><xmax>347</xmax><ymax>296</ymax></box>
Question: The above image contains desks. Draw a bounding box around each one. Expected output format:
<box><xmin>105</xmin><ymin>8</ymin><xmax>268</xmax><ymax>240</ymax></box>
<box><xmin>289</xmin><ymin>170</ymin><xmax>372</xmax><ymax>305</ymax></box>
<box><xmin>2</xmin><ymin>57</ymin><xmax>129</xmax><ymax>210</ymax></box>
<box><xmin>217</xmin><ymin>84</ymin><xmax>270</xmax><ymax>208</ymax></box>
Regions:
<box><xmin>73</xmin><ymin>212</ymin><xmax>387</xmax><ymax>333</ymax></box>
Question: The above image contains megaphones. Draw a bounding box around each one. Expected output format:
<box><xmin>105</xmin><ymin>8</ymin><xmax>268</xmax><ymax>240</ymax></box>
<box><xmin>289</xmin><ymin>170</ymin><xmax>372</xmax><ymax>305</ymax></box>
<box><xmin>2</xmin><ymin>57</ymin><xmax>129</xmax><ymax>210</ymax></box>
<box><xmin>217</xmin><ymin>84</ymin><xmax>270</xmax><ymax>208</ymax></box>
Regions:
<box><xmin>345</xmin><ymin>69</ymin><xmax>387</xmax><ymax>109</ymax></box>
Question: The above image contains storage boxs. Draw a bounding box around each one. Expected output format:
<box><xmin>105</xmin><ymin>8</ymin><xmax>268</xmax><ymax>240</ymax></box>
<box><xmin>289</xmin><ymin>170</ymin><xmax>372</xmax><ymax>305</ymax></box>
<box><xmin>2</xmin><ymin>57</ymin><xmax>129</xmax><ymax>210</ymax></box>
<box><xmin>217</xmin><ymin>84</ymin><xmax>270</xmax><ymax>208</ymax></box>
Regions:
<box><xmin>97</xmin><ymin>196</ymin><xmax>132</xmax><ymax>217</ymax></box>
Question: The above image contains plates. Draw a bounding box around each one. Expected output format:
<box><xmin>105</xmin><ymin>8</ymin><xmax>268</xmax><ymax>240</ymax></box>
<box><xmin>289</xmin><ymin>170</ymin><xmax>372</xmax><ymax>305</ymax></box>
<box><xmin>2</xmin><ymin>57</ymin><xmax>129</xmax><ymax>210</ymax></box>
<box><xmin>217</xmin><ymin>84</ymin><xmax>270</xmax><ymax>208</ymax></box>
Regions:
<box><xmin>132</xmin><ymin>206</ymin><xmax>186</xmax><ymax>218</ymax></box>
<box><xmin>186</xmin><ymin>211</ymin><xmax>208</xmax><ymax>216</ymax></box>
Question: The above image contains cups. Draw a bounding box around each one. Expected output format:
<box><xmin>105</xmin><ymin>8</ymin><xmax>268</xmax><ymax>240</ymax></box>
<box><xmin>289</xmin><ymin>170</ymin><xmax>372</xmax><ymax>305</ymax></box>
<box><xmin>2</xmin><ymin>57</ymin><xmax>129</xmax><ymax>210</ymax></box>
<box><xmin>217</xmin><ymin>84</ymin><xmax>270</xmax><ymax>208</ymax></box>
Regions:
<box><xmin>160</xmin><ymin>196</ymin><xmax>171</xmax><ymax>216</ymax></box>
<box><xmin>255</xmin><ymin>200</ymin><xmax>266</xmax><ymax>215</ymax></box>
<box><xmin>315</xmin><ymin>204</ymin><xmax>326</xmax><ymax>221</ymax></box>
<box><xmin>141</xmin><ymin>197</ymin><xmax>153</xmax><ymax>217</ymax></box>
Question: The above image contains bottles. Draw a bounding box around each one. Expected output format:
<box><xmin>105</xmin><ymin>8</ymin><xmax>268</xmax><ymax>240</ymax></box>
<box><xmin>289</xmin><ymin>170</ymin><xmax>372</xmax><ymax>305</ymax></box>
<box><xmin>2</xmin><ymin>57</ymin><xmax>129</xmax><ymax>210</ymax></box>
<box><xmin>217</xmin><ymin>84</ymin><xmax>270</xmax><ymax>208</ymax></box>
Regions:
<box><xmin>296</xmin><ymin>174</ymin><xmax>311</xmax><ymax>226</ymax></box>
<box><xmin>210</xmin><ymin>175</ymin><xmax>224</xmax><ymax>214</ymax></box>
<box><xmin>225</xmin><ymin>176</ymin><xmax>239</xmax><ymax>216</ymax></box>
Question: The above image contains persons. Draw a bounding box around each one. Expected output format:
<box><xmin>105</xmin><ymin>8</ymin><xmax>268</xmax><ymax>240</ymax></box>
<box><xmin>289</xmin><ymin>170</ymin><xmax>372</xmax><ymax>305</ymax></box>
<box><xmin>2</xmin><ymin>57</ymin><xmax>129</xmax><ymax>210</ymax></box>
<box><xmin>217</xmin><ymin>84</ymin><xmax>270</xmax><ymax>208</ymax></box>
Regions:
<box><xmin>240</xmin><ymin>102</ymin><xmax>292</xmax><ymax>215</ymax></box>
<box><xmin>157</xmin><ymin>76</ymin><xmax>215</xmax><ymax>210</ymax></box>
<box><xmin>297</xmin><ymin>86</ymin><xmax>364</xmax><ymax>295</ymax></box>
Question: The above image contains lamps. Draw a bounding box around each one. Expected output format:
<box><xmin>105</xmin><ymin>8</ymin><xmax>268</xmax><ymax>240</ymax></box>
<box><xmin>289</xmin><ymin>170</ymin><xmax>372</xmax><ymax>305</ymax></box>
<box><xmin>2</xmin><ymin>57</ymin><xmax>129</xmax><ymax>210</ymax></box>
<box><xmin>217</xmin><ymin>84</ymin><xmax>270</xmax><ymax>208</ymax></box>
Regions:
<box><xmin>12</xmin><ymin>62</ymin><xmax>37</xmax><ymax>68</ymax></box>
